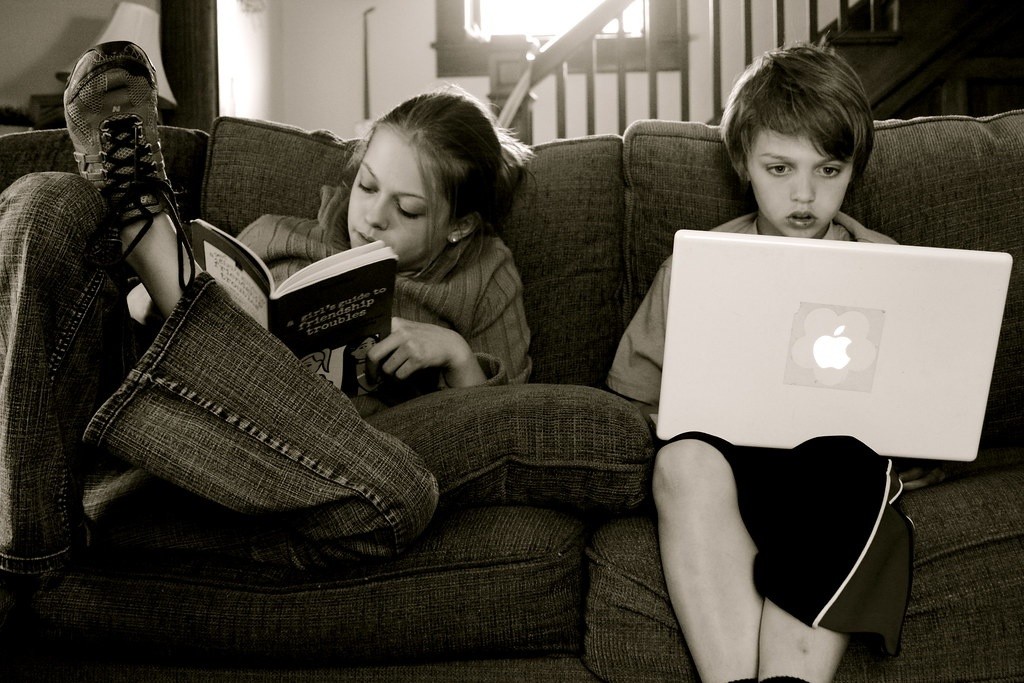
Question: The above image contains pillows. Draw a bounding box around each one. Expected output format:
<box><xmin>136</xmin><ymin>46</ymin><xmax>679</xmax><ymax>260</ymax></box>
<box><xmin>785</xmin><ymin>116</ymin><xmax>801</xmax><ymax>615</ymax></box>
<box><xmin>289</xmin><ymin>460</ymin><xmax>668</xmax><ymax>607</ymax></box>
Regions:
<box><xmin>201</xmin><ymin>117</ymin><xmax>627</xmax><ymax>406</ymax></box>
<box><xmin>0</xmin><ymin>127</ymin><xmax>209</xmax><ymax>229</ymax></box>
<box><xmin>362</xmin><ymin>381</ymin><xmax>656</xmax><ymax>511</ymax></box>
<box><xmin>622</xmin><ymin>108</ymin><xmax>1023</xmax><ymax>437</ymax></box>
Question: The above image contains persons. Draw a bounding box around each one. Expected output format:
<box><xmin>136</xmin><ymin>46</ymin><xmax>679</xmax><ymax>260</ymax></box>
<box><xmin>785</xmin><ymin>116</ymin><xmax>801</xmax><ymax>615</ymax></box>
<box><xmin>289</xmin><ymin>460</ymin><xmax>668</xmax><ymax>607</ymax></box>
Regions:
<box><xmin>0</xmin><ymin>40</ymin><xmax>532</xmax><ymax>575</ymax></box>
<box><xmin>606</xmin><ymin>47</ymin><xmax>946</xmax><ymax>683</ymax></box>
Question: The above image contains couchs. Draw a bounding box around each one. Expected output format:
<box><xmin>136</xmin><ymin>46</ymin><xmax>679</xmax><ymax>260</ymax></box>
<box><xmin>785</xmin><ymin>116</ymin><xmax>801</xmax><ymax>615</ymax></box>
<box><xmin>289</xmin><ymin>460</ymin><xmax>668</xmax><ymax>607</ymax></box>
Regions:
<box><xmin>0</xmin><ymin>437</ymin><xmax>1023</xmax><ymax>682</ymax></box>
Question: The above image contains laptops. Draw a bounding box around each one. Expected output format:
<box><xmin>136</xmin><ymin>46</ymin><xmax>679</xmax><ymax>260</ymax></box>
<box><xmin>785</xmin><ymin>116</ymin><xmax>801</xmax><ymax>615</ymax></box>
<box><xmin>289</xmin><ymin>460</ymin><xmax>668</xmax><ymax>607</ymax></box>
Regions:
<box><xmin>657</xmin><ymin>229</ymin><xmax>1013</xmax><ymax>462</ymax></box>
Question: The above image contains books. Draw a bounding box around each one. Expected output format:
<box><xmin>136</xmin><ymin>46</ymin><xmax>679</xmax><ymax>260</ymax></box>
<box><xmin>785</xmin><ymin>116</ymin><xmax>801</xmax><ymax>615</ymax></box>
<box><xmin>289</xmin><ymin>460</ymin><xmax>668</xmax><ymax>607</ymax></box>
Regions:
<box><xmin>189</xmin><ymin>217</ymin><xmax>399</xmax><ymax>399</ymax></box>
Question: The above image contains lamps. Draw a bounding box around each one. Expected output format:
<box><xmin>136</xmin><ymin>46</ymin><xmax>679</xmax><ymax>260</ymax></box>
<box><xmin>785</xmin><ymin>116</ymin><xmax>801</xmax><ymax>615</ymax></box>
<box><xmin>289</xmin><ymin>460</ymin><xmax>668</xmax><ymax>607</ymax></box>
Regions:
<box><xmin>55</xmin><ymin>1</ymin><xmax>176</xmax><ymax>110</ymax></box>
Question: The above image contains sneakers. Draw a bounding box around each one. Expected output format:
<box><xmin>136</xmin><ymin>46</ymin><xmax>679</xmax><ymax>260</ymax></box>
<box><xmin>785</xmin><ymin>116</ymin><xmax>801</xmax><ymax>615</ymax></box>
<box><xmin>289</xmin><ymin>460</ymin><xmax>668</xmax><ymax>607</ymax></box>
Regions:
<box><xmin>62</xmin><ymin>40</ymin><xmax>197</xmax><ymax>293</ymax></box>
